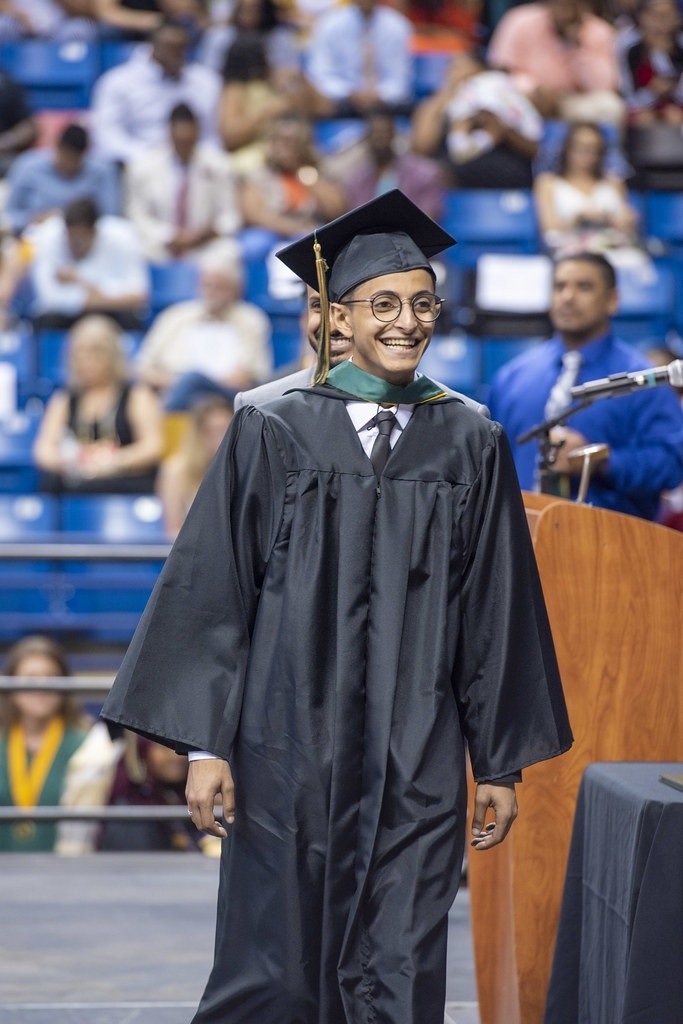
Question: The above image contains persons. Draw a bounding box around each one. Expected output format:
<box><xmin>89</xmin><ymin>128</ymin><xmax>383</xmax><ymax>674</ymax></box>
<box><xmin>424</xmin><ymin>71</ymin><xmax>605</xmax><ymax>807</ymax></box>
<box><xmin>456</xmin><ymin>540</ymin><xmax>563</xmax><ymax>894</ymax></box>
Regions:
<box><xmin>94</xmin><ymin>189</ymin><xmax>576</xmax><ymax>1024</ymax></box>
<box><xmin>0</xmin><ymin>1</ymin><xmax>683</xmax><ymax>862</ymax></box>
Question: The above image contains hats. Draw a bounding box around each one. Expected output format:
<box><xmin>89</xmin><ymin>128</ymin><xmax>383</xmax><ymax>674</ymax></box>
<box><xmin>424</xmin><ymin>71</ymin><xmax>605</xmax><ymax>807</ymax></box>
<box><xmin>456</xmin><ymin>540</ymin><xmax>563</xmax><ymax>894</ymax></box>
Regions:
<box><xmin>274</xmin><ymin>187</ymin><xmax>458</xmax><ymax>305</ymax></box>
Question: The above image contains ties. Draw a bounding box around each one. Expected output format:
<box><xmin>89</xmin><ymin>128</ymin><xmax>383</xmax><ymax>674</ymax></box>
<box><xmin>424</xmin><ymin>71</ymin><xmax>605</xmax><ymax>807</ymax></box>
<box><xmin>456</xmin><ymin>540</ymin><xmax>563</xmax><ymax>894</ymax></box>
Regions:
<box><xmin>541</xmin><ymin>350</ymin><xmax>582</xmax><ymax>425</ymax></box>
<box><xmin>371</xmin><ymin>411</ymin><xmax>401</xmax><ymax>479</ymax></box>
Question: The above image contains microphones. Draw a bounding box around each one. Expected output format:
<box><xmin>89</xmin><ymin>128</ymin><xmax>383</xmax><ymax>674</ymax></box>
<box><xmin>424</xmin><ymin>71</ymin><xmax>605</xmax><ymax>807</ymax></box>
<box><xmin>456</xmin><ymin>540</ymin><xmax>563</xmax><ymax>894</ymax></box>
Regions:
<box><xmin>571</xmin><ymin>359</ymin><xmax>682</xmax><ymax>397</ymax></box>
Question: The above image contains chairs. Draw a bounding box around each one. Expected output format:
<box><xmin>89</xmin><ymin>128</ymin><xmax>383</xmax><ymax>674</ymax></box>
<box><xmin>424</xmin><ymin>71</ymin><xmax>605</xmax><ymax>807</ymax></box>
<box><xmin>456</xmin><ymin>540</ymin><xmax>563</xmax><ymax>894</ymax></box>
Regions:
<box><xmin>3</xmin><ymin>0</ymin><xmax>678</xmax><ymax>645</ymax></box>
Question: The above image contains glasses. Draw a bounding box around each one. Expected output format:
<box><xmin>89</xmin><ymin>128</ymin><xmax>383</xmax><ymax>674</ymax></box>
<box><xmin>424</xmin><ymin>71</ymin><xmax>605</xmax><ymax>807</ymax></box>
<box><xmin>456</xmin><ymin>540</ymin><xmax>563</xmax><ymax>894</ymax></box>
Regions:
<box><xmin>340</xmin><ymin>292</ymin><xmax>446</xmax><ymax>323</ymax></box>
